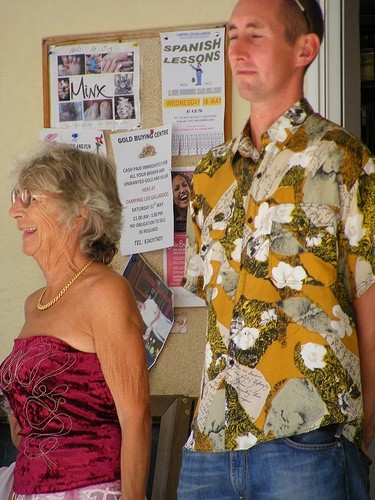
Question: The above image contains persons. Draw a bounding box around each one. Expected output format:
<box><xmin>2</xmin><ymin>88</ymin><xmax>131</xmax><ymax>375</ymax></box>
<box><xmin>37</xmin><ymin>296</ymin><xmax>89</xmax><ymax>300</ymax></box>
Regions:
<box><xmin>0</xmin><ymin>143</ymin><xmax>151</xmax><ymax>500</ymax></box>
<box><xmin>58</xmin><ymin>52</ymin><xmax>135</xmax><ymax>121</ymax></box>
<box><xmin>136</xmin><ymin>299</ymin><xmax>172</xmax><ymax>367</ymax></box>
<box><xmin>172</xmin><ymin>172</ymin><xmax>191</xmax><ymax>221</ymax></box>
<box><xmin>0</xmin><ymin>397</ymin><xmax>22</xmax><ymax>500</ymax></box>
<box><xmin>177</xmin><ymin>0</ymin><xmax>375</xmax><ymax>500</ymax></box>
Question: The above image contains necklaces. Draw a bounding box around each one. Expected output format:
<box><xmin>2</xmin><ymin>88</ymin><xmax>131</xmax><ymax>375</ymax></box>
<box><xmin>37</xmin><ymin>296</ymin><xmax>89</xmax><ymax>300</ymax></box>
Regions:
<box><xmin>37</xmin><ymin>259</ymin><xmax>95</xmax><ymax>310</ymax></box>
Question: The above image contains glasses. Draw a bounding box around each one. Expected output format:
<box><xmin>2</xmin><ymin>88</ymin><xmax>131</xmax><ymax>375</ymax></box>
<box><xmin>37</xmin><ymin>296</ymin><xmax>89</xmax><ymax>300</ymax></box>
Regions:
<box><xmin>11</xmin><ymin>188</ymin><xmax>49</xmax><ymax>208</ymax></box>
